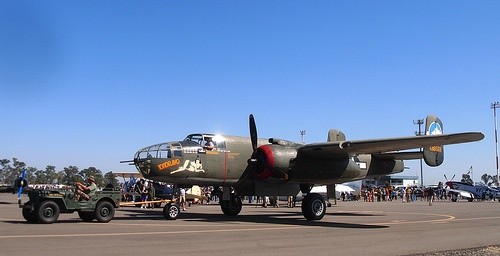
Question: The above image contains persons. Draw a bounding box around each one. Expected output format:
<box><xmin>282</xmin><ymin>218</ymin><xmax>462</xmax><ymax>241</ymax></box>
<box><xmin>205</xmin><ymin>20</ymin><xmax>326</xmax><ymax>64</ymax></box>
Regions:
<box><xmin>200</xmin><ymin>187</ymin><xmax>219</xmax><ymax>205</ymax></box>
<box><xmin>272</xmin><ymin>196</ymin><xmax>280</xmax><ymax>208</ymax></box>
<box><xmin>340</xmin><ymin>191</ymin><xmax>356</xmax><ymax>201</ymax></box>
<box><xmin>287</xmin><ymin>196</ymin><xmax>293</xmax><ymax>208</ymax></box>
<box><xmin>480</xmin><ymin>191</ymin><xmax>497</xmax><ymax>202</ymax></box>
<box><xmin>365</xmin><ymin>185</ymin><xmax>399</xmax><ymax>202</ymax></box>
<box><xmin>263</xmin><ymin>195</ymin><xmax>268</xmax><ymax>208</ymax></box>
<box><xmin>202</xmin><ymin>137</ymin><xmax>214</xmax><ymax>150</ymax></box>
<box><xmin>242</xmin><ymin>195</ymin><xmax>262</xmax><ymax>203</ymax></box>
<box><xmin>179</xmin><ymin>187</ymin><xmax>187</xmax><ymax>211</ymax></box>
<box><xmin>141</xmin><ymin>185</ymin><xmax>148</xmax><ymax>210</ymax></box>
<box><xmin>148</xmin><ymin>183</ymin><xmax>155</xmax><ymax>209</ymax></box>
<box><xmin>399</xmin><ymin>185</ymin><xmax>447</xmax><ymax>206</ymax></box>
<box><xmin>73</xmin><ymin>175</ymin><xmax>98</xmax><ymax>202</ymax></box>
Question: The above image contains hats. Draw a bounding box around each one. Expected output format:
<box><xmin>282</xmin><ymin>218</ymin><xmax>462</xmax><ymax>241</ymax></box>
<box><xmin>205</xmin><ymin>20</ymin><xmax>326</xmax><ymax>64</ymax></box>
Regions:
<box><xmin>86</xmin><ymin>175</ymin><xmax>95</xmax><ymax>182</ymax></box>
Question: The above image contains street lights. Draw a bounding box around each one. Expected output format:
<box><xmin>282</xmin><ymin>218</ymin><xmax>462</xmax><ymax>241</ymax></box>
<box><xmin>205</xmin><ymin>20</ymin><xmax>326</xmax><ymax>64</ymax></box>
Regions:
<box><xmin>300</xmin><ymin>130</ymin><xmax>306</xmax><ymax>142</ymax></box>
<box><xmin>412</xmin><ymin>118</ymin><xmax>425</xmax><ymax>185</ymax></box>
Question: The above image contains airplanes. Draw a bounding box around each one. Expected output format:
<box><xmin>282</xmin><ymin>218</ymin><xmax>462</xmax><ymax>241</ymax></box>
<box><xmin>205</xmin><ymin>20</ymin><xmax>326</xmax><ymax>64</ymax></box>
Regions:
<box><xmin>441</xmin><ymin>174</ymin><xmax>500</xmax><ymax>202</ymax></box>
<box><xmin>133</xmin><ymin>114</ymin><xmax>486</xmax><ymax>221</ymax></box>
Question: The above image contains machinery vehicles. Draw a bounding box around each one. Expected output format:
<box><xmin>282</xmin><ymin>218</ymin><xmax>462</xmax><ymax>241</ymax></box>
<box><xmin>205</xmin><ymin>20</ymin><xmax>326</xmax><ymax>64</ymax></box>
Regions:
<box><xmin>23</xmin><ymin>185</ymin><xmax>181</xmax><ymax>222</ymax></box>
